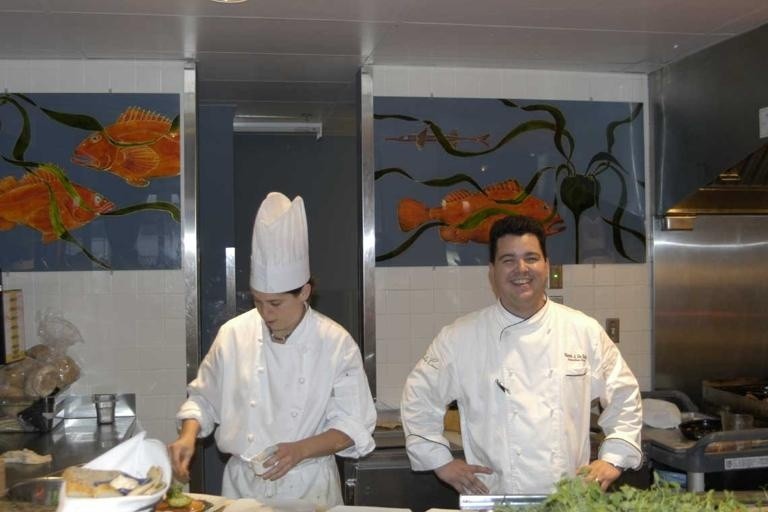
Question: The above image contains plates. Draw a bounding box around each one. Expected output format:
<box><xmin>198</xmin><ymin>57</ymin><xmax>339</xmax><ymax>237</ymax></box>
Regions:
<box><xmin>155</xmin><ymin>492</ymin><xmax>228</xmax><ymax>512</ymax></box>
<box><xmin>55</xmin><ymin>437</ymin><xmax>173</xmax><ymax>512</ymax></box>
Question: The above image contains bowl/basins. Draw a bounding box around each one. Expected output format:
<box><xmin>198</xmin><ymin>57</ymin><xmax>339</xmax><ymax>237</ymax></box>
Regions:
<box><xmin>678</xmin><ymin>418</ymin><xmax>727</xmax><ymax>442</ymax></box>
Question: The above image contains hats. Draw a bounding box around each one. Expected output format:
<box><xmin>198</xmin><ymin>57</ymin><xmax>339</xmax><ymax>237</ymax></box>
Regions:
<box><xmin>249</xmin><ymin>189</ymin><xmax>312</xmax><ymax>296</ymax></box>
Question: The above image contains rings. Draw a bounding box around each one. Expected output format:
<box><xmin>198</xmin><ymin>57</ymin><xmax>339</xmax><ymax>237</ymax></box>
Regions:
<box><xmin>595</xmin><ymin>478</ymin><xmax>601</xmax><ymax>485</ymax></box>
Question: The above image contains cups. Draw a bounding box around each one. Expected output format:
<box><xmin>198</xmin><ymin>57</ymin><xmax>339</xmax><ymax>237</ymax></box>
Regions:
<box><xmin>723</xmin><ymin>413</ymin><xmax>753</xmax><ymax>453</ymax></box>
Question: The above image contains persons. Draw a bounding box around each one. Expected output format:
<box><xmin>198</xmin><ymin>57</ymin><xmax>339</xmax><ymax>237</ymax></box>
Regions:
<box><xmin>400</xmin><ymin>215</ymin><xmax>643</xmax><ymax>496</ymax></box>
<box><xmin>164</xmin><ymin>192</ymin><xmax>378</xmax><ymax>505</ymax></box>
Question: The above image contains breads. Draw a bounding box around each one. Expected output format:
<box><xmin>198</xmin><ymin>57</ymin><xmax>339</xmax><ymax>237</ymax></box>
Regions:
<box><xmin>66</xmin><ymin>482</ymin><xmax>122</xmax><ymax>497</ymax></box>
<box><xmin>0</xmin><ymin>344</ymin><xmax>79</xmax><ymax>398</ymax></box>
